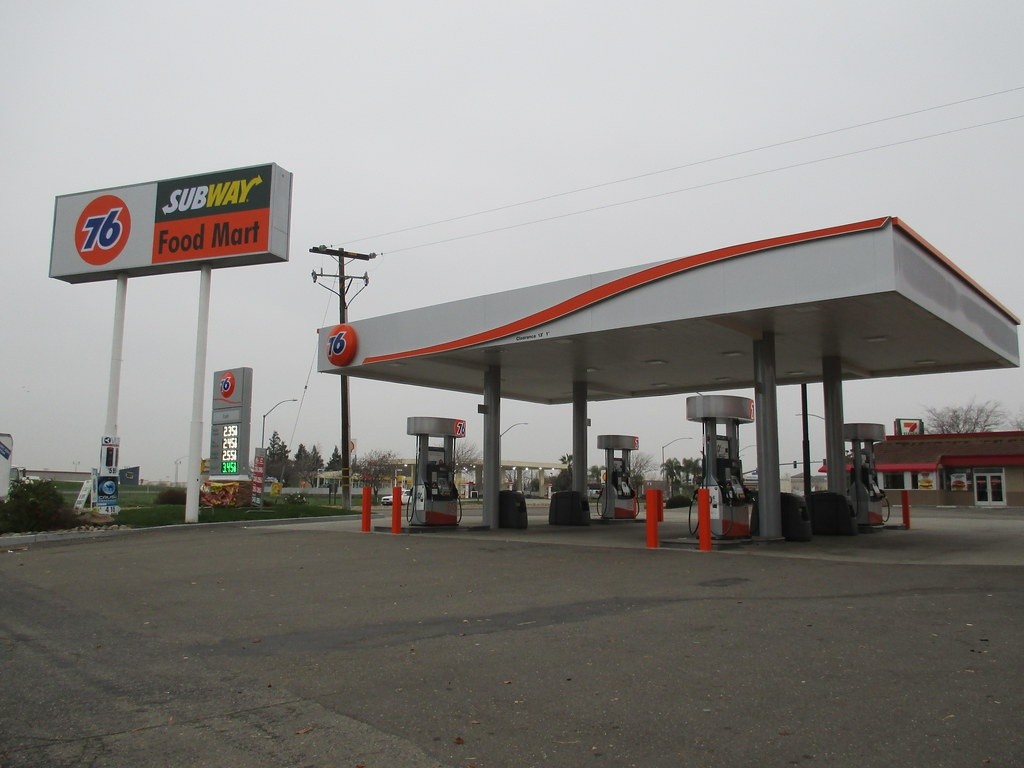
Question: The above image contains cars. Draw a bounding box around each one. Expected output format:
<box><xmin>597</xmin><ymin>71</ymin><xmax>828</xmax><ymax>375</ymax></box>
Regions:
<box><xmin>381</xmin><ymin>490</ymin><xmax>412</xmax><ymax>506</ymax></box>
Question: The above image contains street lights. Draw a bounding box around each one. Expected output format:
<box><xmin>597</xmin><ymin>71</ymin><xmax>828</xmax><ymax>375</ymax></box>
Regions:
<box><xmin>662</xmin><ymin>437</ymin><xmax>693</xmax><ymax>480</ymax></box>
<box><xmin>262</xmin><ymin>399</ymin><xmax>297</xmax><ymax>449</ymax></box>
<box><xmin>500</xmin><ymin>423</ymin><xmax>528</xmax><ymax>466</ymax></box>
